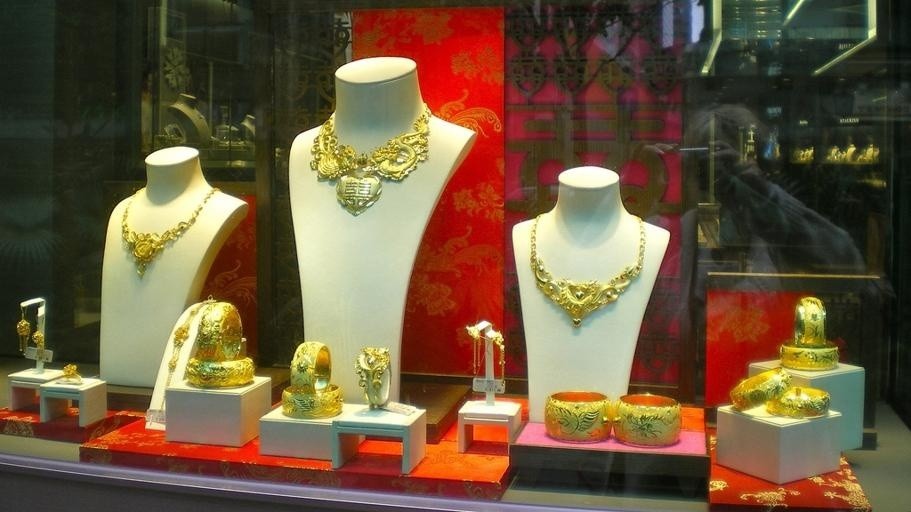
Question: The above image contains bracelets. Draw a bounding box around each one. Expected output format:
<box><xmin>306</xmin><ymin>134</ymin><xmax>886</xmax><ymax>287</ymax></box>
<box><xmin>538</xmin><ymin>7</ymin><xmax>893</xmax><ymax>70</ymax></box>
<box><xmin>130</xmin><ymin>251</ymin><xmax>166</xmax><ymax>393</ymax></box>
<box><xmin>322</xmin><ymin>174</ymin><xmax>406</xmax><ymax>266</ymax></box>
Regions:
<box><xmin>357</xmin><ymin>344</ymin><xmax>392</xmax><ymax>408</ymax></box>
<box><xmin>544</xmin><ymin>390</ymin><xmax>614</xmax><ymax>445</ymax></box>
<box><xmin>152</xmin><ymin>294</ymin><xmax>255</xmax><ymax>414</ymax></box>
<box><xmin>615</xmin><ymin>393</ymin><xmax>683</xmax><ymax>446</ymax></box>
<box><xmin>729</xmin><ymin>296</ymin><xmax>841</xmax><ymax>419</ymax></box>
<box><xmin>281</xmin><ymin>384</ymin><xmax>344</xmax><ymax>421</ymax></box>
<box><xmin>289</xmin><ymin>340</ymin><xmax>332</xmax><ymax>393</ymax></box>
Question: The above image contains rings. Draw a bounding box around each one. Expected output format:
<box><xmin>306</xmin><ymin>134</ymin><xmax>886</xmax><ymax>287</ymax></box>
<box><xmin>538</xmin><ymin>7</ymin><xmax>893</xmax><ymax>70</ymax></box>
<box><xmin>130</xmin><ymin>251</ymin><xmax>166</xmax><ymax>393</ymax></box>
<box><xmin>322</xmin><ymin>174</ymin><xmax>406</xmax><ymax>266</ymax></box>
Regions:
<box><xmin>63</xmin><ymin>363</ymin><xmax>83</xmax><ymax>384</ymax></box>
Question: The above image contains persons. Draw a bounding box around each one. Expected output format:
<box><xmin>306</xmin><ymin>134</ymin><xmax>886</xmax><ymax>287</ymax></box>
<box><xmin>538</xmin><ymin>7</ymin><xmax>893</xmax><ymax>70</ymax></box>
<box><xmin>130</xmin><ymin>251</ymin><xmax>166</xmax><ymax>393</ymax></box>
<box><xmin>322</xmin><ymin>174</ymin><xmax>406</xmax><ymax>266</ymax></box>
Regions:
<box><xmin>636</xmin><ymin>104</ymin><xmax>898</xmax><ymax>409</ymax></box>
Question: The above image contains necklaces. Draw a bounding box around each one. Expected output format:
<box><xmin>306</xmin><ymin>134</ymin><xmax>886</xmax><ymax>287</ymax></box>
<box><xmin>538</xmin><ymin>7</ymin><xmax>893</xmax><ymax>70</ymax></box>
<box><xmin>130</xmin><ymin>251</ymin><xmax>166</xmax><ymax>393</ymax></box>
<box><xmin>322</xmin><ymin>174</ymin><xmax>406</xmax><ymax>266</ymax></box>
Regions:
<box><xmin>308</xmin><ymin>105</ymin><xmax>431</xmax><ymax>216</ymax></box>
<box><xmin>121</xmin><ymin>185</ymin><xmax>220</xmax><ymax>278</ymax></box>
<box><xmin>530</xmin><ymin>213</ymin><xmax>646</xmax><ymax>328</ymax></box>
<box><xmin>176</xmin><ymin>101</ymin><xmax>206</xmax><ymax>122</ymax></box>
